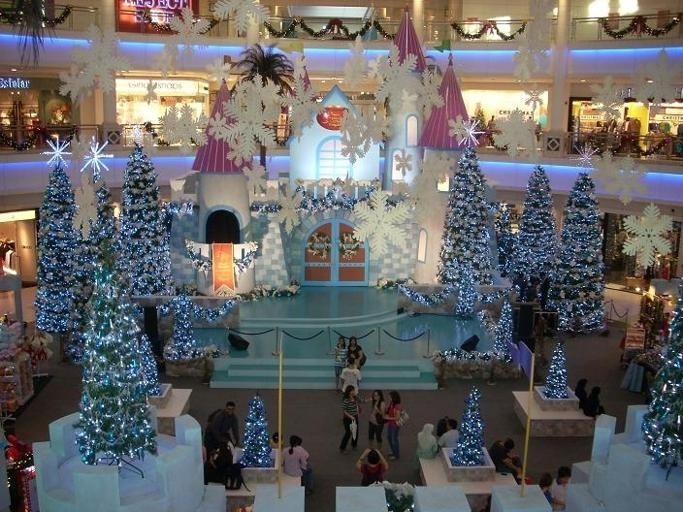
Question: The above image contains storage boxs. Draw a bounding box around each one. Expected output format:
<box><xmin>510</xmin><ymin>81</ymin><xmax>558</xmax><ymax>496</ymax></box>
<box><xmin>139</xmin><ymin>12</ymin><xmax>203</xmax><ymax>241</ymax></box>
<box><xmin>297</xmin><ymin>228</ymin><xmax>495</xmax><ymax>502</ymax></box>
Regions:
<box><xmin>624</xmin><ymin>326</ymin><xmax>645</xmax><ymax>350</ymax></box>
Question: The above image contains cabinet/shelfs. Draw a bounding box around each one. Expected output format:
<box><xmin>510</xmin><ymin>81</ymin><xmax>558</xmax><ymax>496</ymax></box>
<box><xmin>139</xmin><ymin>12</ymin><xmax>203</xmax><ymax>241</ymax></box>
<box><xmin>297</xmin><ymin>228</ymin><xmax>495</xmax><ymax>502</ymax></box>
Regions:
<box><xmin>0</xmin><ymin>352</ymin><xmax>34</xmax><ymax>422</ymax></box>
<box><xmin>639</xmin><ymin>289</ymin><xmax>663</xmax><ymax>347</ymax></box>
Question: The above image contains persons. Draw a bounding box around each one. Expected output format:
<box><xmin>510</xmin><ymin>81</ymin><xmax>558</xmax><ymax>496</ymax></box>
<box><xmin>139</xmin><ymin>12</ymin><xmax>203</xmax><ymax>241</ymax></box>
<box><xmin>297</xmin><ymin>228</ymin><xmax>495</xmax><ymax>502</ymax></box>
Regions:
<box><xmin>540</xmin><ymin>466</ymin><xmax>572</xmax><ymax>512</ymax></box>
<box><xmin>356</xmin><ymin>449</ymin><xmax>389</xmax><ymax>486</ymax></box>
<box><xmin>575</xmin><ymin>378</ymin><xmax>603</xmax><ymax>418</ymax></box>
<box><xmin>579</xmin><ymin>118</ymin><xmax>657</xmax><ymax>160</ymax></box>
<box><xmin>339</xmin><ymin>386</ymin><xmax>405</xmax><ymax>461</ymax></box>
<box><xmin>334</xmin><ymin>337</ymin><xmax>363</xmax><ymax>395</ymax></box>
<box><xmin>200</xmin><ymin>401</ymin><xmax>311</xmax><ymax>496</ymax></box>
<box><xmin>489</xmin><ymin>437</ymin><xmax>523</xmax><ymax>485</ymax></box>
<box><xmin>414</xmin><ymin>416</ymin><xmax>458</xmax><ymax>460</ymax></box>
